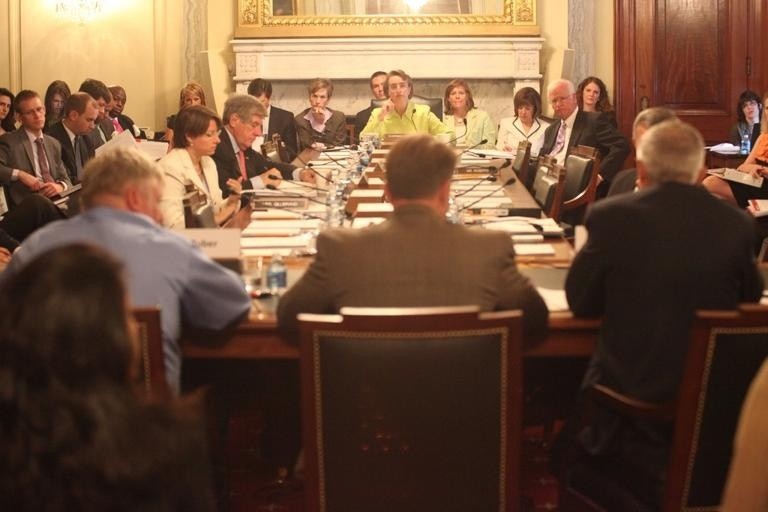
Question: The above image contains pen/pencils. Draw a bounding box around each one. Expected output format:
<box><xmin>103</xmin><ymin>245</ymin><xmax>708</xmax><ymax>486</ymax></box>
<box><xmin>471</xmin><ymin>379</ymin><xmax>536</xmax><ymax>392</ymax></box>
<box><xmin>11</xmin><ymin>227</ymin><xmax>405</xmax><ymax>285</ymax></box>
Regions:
<box><xmin>752</xmin><ymin>201</ymin><xmax>760</xmax><ymax>211</ymax></box>
<box><xmin>252</xmin><ymin>210</ymin><xmax>267</xmax><ymax>211</ymax></box>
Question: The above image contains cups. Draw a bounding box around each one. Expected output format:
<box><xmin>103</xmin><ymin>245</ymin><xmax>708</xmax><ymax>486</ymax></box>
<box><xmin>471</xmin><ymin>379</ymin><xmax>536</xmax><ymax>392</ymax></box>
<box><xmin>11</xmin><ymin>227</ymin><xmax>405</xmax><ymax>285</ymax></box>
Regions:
<box><xmin>242</xmin><ymin>255</ymin><xmax>263</xmax><ymax>295</ymax></box>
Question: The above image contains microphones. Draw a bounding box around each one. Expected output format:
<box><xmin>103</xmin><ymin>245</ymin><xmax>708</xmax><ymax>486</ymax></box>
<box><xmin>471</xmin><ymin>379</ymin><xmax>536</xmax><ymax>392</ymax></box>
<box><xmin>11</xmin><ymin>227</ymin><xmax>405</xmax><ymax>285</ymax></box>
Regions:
<box><xmin>410</xmin><ymin>106</ymin><xmax>422</xmax><ymax>135</ymax></box>
<box><xmin>450</xmin><ymin>117</ymin><xmax>518</xmax><ymax>211</ymax></box>
<box><xmin>221</xmin><ymin>124</ymin><xmax>361</xmax><ymax>223</ymax></box>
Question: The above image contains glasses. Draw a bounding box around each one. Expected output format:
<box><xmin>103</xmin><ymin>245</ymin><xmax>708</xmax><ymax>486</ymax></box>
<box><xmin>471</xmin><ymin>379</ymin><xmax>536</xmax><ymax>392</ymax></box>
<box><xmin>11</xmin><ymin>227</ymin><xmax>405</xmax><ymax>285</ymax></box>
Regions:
<box><xmin>200</xmin><ymin>130</ymin><xmax>223</xmax><ymax>137</ymax></box>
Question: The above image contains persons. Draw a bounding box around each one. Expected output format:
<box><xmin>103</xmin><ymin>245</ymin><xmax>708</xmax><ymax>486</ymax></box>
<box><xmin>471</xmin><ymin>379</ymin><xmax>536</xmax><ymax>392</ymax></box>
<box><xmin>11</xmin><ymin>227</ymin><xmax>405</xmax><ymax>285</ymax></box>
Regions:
<box><xmin>498</xmin><ymin>76</ymin><xmax>675</xmax><ymax>199</ymax></box>
<box><xmin>157</xmin><ymin>77</ymin><xmax>347</xmax><ymax>228</ymax></box>
<box><xmin>275</xmin><ymin>131</ymin><xmax>550</xmax><ymax>354</ymax></box>
<box><xmin>702</xmin><ymin>91</ymin><xmax>768</xmax><ymax>236</ymax></box>
<box><xmin>564</xmin><ymin>121</ymin><xmax>764</xmax><ymax>423</ymax></box>
<box><xmin>353</xmin><ymin>71</ymin><xmax>498</xmax><ymax>151</ymax></box>
<box><xmin>0</xmin><ymin>241</ymin><xmax>219</xmax><ymax>509</ymax></box>
<box><xmin>2</xmin><ymin>146</ymin><xmax>250</xmax><ymax>405</ymax></box>
<box><xmin>1</xmin><ymin>79</ymin><xmax>147</xmax><ymax>260</ymax></box>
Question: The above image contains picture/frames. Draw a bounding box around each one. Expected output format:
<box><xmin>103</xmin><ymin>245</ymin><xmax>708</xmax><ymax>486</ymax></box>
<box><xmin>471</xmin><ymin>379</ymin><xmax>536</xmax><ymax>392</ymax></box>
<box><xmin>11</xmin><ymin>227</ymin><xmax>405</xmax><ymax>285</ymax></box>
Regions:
<box><xmin>232</xmin><ymin>0</ymin><xmax>541</xmax><ymax>39</ymax></box>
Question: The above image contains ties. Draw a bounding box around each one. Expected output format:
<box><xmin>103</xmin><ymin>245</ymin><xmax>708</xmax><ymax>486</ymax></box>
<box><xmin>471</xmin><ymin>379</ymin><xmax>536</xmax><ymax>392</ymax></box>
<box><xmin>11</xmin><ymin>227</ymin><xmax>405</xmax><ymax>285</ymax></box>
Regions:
<box><xmin>74</xmin><ymin>135</ymin><xmax>86</xmax><ymax>180</ymax></box>
<box><xmin>110</xmin><ymin>119</ymin><xmax>124</xmax><ymax>134</ymax></box>
<box><xmin>550</xmin><ymin>121</ymin><xmax>566</xmax><ymax>159</ymax></box>
<box><xmin>238</xmin><ymin>150</ymin><xmax>247</xmax><ymax>181</ymax></box>
<box><xmin>34</xmin><ymin>138</ymin><xmax>55</xmax><ymax>183</ymax></box>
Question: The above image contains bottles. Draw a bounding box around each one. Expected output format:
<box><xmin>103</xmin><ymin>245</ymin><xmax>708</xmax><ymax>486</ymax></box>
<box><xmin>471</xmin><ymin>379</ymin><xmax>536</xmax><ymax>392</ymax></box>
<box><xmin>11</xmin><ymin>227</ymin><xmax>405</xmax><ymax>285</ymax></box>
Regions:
<box><xmin>443</xmin><ymin>196</ymin><xmax>463</xmax><ymax>229</ymax></box>
<box><xmin>740</xmin><ymin>133</ymin><xmax>752</xmax><ymax>158</ymax></box>
<box><xmin>268</xmin><ymin>249</ymin><xmax>289</xmax><ymax>318</ymax></box>
<box><xmin>325</xmin><ymin>132</ymin><xmax>381</xmax><ymax>236</ymax></box>
